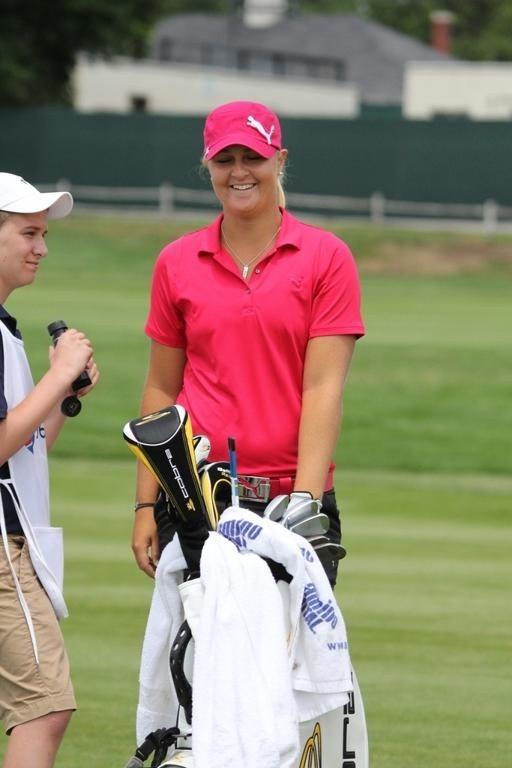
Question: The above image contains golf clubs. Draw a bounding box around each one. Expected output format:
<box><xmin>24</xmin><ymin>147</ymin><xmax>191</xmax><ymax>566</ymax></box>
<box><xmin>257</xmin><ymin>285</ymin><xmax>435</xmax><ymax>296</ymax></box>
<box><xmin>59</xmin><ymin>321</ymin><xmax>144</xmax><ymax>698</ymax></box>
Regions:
<box><xmin>122</xmin><ymin>404</ymin><xmax>347</xmax><ymax>563</ymax></box>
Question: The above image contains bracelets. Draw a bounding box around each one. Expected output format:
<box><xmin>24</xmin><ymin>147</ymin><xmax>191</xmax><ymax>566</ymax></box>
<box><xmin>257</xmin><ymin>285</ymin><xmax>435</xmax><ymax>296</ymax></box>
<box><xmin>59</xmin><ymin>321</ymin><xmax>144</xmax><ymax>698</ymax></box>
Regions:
<box><xmin>135</xmin><ymin>501</ymin><xmax>157</xmax><ymax>511</ymax></box>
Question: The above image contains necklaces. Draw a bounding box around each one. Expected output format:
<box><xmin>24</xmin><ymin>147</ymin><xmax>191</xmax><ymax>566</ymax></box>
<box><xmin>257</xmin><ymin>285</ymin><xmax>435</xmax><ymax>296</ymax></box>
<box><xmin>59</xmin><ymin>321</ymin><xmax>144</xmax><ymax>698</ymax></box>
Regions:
<box><xmin>217</xmin><ymin>214</ymin><xmax>282</xmax><ymax>277</ymax></box>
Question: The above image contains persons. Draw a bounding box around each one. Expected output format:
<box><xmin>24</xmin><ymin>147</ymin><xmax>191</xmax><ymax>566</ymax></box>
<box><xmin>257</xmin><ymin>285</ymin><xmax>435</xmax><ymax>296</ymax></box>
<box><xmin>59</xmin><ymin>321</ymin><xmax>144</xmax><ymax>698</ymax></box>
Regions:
<box><xmin>2</xmin><ymin>169</ymin><xmax>102</xmax><ymax>767</ymax></box>
<box><xmin>135</xmin><ymin>100</ymin><xmax>366</xmax><ymax>589</ymax></box>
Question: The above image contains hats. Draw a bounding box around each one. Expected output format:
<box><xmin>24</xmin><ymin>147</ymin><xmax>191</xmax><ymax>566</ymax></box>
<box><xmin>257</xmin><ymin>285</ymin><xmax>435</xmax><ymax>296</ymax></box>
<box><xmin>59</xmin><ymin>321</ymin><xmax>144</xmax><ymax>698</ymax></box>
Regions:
<box><xmin>0</xmin><ymin>172</ymin><xmax>75</xmax><ymax>221</ymax></box>
<box><xmin>203</xmin><ymin>100</ymin><xmax>282</xmax><ymax>161</ymax></box>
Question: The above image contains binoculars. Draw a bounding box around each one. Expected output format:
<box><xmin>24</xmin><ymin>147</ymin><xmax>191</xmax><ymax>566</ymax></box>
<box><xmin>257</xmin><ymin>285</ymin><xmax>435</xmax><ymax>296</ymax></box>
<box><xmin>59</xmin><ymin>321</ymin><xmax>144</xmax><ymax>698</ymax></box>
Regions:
<box><xmin>48</xmin><ymin>320</ymin><xmax>92</xmax><ymax>392</ymax></box>
